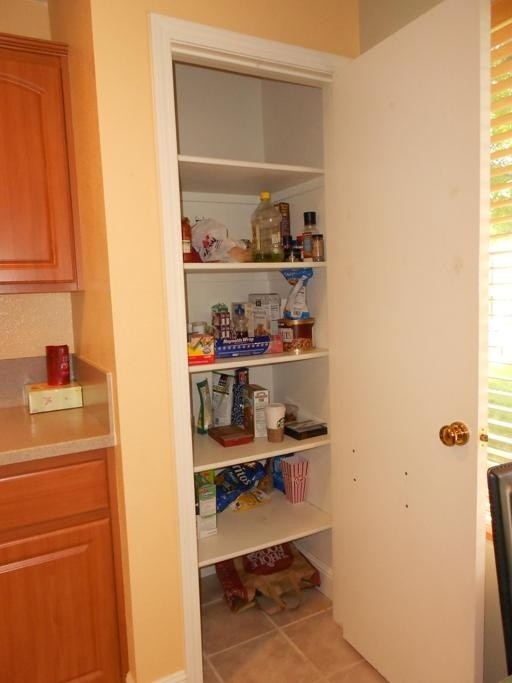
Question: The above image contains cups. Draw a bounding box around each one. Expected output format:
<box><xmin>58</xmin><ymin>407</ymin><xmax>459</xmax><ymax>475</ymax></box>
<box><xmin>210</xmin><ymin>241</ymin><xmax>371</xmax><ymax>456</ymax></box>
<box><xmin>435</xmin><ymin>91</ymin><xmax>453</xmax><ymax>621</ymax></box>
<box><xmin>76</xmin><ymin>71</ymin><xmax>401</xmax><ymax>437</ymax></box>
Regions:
<box><xmin>264</xmin><ymin>403</ymin><xmax>286</xmax><ymax>443</ymax></box>
<box><xmin>285</xmin><ymin>404</ymin><xmax>299</xmax><ymax>423</ymax></box>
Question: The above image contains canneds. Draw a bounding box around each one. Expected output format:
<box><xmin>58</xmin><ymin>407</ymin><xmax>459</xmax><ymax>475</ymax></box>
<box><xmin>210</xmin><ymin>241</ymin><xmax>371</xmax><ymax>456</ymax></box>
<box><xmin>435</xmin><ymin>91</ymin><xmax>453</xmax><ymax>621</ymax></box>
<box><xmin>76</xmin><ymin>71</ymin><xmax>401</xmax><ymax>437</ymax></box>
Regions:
<box><xmin>311</xmin><ymin>234</ymin><xmax>324</xmax><ymax>262</ymax></box>
<box><xmin>297</xmin><ymin>236</ymin><xmax>304</xmax><ymax>262</ymax></box>
<box><xmin>187</xmin><ymin>322</ymin><xmax>207</xmax><ymax>344</ymax></box>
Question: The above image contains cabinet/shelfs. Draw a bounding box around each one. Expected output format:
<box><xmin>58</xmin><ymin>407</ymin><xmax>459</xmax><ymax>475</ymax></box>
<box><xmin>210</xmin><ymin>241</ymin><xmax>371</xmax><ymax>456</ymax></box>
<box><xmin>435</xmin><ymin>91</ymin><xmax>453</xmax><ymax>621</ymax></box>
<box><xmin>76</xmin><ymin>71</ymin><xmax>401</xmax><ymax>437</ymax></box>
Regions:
<box><xmin>0</xmin><ymin>448</ymin><xmax>130</xmax><ymax>683</ymax></box>
<box><xmin>171</xmin><ymin>36</ymin><xmax>339</xmax><ymax>655</ymax></box>
<box><xmin>0</xmin><ymin>32</ymin><xmax>86</xmax><ymax>294</ymax></box>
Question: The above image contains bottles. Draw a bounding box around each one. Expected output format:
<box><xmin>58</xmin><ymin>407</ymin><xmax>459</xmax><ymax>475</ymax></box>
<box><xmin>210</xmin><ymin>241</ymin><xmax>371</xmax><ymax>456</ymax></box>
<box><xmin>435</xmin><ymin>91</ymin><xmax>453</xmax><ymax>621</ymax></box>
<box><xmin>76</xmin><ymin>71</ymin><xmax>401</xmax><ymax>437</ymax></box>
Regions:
<box><xmin>251</xmin><ymin>189</ymin><xmax>325</xmax><ymax>263</ymax></box>
<box><xmin>181</xmin><ymin>214</ymin><xmax>193</xmax><ymax>263</ymax></box>
<box><xmin>232</xmin><ymin>300</ymin><xmax>316</xmax><ymax>355</ymax></box>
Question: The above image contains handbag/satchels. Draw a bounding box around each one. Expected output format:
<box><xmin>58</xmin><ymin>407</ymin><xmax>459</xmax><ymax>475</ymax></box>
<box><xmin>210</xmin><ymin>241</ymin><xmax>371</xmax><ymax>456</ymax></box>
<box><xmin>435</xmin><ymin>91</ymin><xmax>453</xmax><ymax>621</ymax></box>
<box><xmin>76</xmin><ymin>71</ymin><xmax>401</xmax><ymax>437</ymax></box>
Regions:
<box><xmin>214</xmin><ymin>542</ymin><xmax>322</xmax><ymax>617</ymax></box>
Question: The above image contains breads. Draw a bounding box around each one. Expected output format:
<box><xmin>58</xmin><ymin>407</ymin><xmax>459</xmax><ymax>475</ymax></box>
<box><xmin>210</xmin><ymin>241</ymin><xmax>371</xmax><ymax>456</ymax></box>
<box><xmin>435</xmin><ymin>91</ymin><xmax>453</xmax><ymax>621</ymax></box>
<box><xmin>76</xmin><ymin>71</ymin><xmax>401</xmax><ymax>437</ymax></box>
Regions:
<box><xmin>227</xmin><ymin>247</ymin><xmax>252</xmax><ymax>262</ymax></box>
